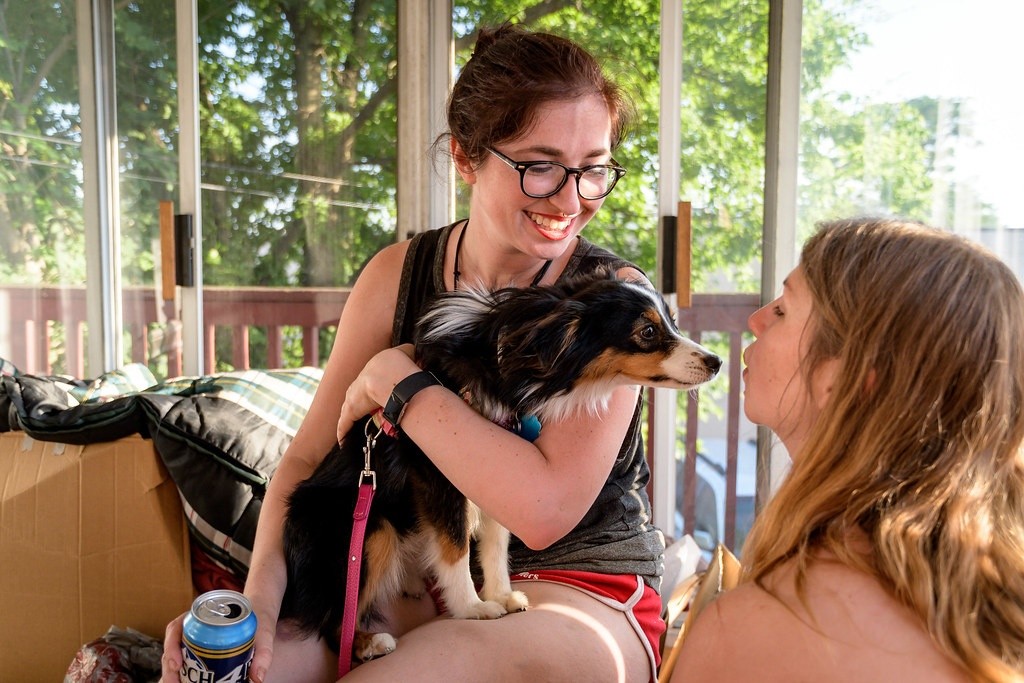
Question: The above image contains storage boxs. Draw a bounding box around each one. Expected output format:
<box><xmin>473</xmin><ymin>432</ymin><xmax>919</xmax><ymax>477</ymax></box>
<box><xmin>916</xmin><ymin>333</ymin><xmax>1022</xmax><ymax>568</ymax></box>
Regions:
<box><xmin>0</xmin><ymin>429</ymin><xmax>193</xmax><ymax>683</ymax></box>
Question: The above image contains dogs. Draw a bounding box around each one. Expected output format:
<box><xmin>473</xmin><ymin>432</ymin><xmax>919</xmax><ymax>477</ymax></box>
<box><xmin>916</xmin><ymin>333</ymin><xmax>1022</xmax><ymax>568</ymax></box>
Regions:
<box><xmin>281</xmin><ymin>261</ymin><xmax>724</xmax><ymax>664</ymax></box>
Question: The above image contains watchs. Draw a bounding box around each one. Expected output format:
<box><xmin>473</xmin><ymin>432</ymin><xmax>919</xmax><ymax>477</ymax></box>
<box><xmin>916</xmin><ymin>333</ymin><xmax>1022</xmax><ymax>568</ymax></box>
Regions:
<box><xmin>382</xmin><ymin>370</ymin><xmax>445</xmax><ymax>427</ymax></box>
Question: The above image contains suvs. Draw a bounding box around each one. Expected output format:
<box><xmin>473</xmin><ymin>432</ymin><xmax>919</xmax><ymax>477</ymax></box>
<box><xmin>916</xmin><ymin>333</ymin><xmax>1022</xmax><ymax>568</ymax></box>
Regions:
<box><xmin>674</xmin><ymin>426</ymin><xmax>792</xmax><ymax>584</ymax></box>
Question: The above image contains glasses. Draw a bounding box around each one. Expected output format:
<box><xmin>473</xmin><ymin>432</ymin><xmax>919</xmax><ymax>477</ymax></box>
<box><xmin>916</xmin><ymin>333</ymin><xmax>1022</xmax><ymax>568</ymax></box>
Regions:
<box><xmin>480</xmin><ymin>143</ymin><xmax>627</xmax><ymax>200</ymax></box>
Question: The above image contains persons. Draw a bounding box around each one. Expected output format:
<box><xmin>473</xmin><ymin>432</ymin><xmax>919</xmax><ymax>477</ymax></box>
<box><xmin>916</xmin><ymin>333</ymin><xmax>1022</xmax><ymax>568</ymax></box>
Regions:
<box><xmin>159</xmin><ymin>20</ymin><xmax>668</xmax><ymax>683</ymax></box>
<box><xmin>656</xmin><ymin>213</ymin><xmax>1024</xmax><ymax>683</ymax></box>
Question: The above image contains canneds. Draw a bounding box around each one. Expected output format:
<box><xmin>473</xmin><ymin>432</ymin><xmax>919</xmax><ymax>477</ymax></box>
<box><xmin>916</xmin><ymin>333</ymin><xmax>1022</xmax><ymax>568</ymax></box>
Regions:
<box><xmin>177</xmin><ymin>589</ymin><xmax>258</xmax><ymax>683</ymax></box>
